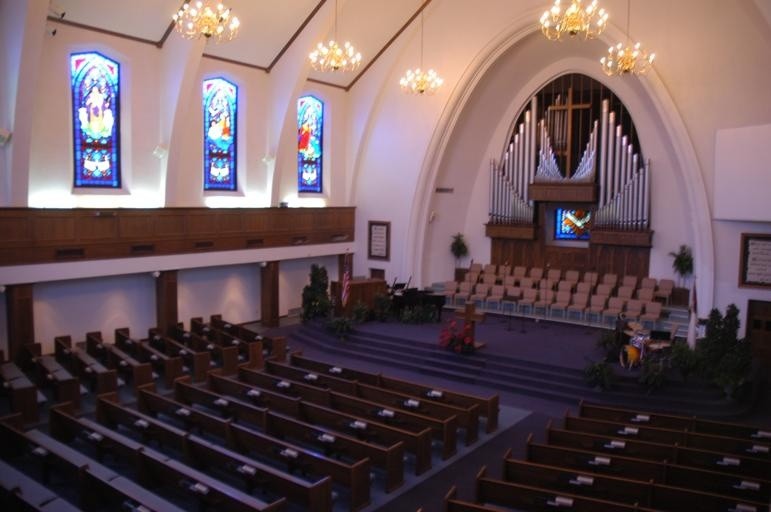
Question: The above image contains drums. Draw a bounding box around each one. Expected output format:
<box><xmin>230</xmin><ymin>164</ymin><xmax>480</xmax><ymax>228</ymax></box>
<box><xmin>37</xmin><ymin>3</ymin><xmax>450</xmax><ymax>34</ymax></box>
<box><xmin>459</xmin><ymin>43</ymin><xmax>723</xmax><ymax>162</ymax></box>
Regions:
<box><xmin>620</xmin><ymin>345</ymin><xmax>640</xmax><ymax>368</ymax></box>
<box><xmin>636</xmin><ymin>329</ymin><xmax>650</xmax><ymax>343</ymax></box>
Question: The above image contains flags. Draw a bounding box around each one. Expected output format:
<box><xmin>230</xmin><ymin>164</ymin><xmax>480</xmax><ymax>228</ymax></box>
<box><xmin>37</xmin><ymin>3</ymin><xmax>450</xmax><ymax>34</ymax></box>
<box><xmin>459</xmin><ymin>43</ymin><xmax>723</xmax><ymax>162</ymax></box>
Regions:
<box><xmin>341</xmin><ymin>253</ymin><xmax>352</xmax><ymax>308</ymax></box>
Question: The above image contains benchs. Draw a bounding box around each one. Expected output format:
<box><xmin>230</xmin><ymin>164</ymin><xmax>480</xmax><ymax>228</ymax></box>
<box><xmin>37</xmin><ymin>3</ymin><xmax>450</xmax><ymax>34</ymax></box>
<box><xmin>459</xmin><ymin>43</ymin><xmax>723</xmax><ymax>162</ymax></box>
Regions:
<box><xmin>0</xmin><ymin>311</ymin><xmax>501</xmax><ymax>512</ymax></box>
<box><xmin>414</xmin><ymin>388</ymin><xmax>771</xmax><ymax>512</ymax></box>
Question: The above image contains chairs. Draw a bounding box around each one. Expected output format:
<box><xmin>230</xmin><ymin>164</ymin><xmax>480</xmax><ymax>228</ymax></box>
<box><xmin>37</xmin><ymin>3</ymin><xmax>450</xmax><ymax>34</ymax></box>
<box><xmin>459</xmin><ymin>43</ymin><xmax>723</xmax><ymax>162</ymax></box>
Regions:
<box><xmin>441</xmin><ymin>261</ymin><xmax>673</xmax><ymax>343</ymax></box>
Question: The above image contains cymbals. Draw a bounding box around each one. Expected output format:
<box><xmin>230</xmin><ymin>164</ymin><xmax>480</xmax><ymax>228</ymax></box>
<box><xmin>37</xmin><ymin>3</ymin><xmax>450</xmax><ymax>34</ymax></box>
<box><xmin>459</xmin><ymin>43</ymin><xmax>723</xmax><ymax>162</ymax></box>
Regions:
<box><xmin>624</xmin><ymin>330</ymin><xmax>638</xmax><ymax>336</ymax></box>
<box><xmin>629</xmin><ymin>322</ymin><xmax>644</xmax><ymax>330</ymax></box>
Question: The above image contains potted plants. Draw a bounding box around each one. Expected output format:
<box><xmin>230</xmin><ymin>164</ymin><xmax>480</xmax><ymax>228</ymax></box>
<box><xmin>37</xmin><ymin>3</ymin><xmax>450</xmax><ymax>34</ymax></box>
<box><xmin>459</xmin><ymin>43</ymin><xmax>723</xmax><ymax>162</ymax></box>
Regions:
<box><xmin>450</xmin><ymin>232</ymin><xmax>471</xmax><ymax>282</ymax></box>
<box><xmin>666</xmin><ymin>244</ymin><xmax>694</xmax><ymax>307</ymax></box>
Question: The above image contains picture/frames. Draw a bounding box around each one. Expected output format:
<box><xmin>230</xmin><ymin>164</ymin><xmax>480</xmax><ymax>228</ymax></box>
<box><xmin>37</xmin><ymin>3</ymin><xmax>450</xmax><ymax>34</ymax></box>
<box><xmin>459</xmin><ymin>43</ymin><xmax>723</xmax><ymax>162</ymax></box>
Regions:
<box><xmin>736</xmin><ymin>230</ymin><xmax>770</xmax><ymax>290</ymax></box>
<box><xmin>368</xmin><ymin>220</ymin><xmax>391</xmax><ymax>260</ymax></box>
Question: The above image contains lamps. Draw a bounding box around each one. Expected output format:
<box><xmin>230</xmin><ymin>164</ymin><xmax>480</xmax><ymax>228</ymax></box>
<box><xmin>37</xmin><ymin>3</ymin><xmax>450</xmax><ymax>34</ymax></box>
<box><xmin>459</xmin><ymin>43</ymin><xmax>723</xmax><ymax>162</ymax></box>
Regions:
<box><xmin>540</xmin><ymin>0</ymin><xmax>656</xmax><ymax>80</ymax></box>
<box><xmin>306</xmin><ymin>4</ymin><xmax>445</xmax><ymax>98</ymax></box>
<box><xmin>173</xmin><ymin>0</ymin><xmax>242</xmax><ymax>41</ymax></box>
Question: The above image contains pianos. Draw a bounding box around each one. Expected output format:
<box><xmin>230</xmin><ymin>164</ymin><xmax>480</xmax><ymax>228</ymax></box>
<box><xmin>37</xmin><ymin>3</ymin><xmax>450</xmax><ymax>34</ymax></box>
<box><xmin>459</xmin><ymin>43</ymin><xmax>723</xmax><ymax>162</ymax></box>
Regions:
<box><xmin>392</xmin><ymin>288</ymin><xmax>447</xmax><ymax>323</ymax></box>
